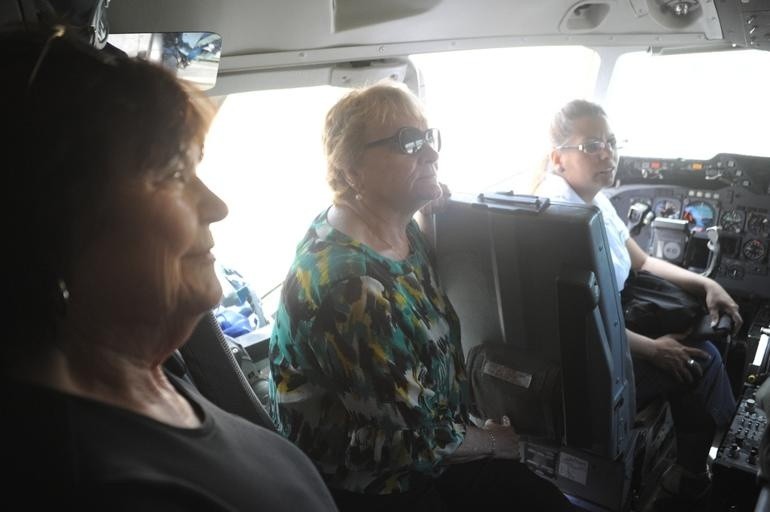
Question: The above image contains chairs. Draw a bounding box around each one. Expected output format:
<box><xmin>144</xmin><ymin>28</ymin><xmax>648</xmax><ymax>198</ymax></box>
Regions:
<box><xmin>424</xmin><ymin>189</ymin><xmax>695</xmax><ymax>512</ymax></box>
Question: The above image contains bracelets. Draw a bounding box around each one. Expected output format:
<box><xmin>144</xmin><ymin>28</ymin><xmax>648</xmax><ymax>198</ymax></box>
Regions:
<box><xmin>487</xmin><ymin>430</ymin><xmax>498</xmax><ymax>456</ymax></box>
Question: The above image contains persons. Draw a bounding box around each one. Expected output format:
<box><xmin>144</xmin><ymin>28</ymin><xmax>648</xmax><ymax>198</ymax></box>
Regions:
<box><xmin>265</xmin><ymin>80</ymin><xmax>583</xmax><ymax>510</ymax></box>
<box><xmin>527</xmin><ymin>96</ymin><xmax>747</xmax><ymax>431</ymax></box>
<box><xmin>0</xmin><ymin>22</ymin><xmax>349</xmax><ymax>511</ymax></box>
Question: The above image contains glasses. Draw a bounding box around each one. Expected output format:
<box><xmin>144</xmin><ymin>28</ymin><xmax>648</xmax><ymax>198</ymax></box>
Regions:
<box><xmin>558</xmin><ymin>138</ymin><xmax>625</xmax><ymax>157</ymax></box>
<box><xmin>366</xmin><ymin>127</ymin><xmax>442</xmax><ymax>156</ymax></box>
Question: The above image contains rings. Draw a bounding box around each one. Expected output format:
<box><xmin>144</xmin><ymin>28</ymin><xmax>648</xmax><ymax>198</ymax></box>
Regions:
<box><xmin>686</xmin><ymin>359</ymin><xmax>696</xmax><ymax>369</ymax></box>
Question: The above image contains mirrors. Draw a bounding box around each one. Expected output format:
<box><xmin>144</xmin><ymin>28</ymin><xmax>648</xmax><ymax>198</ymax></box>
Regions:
<box><xmin>105</xmin><ymin>31</ymin><xmax>222</xmax><ymax>92</ymax></box>
